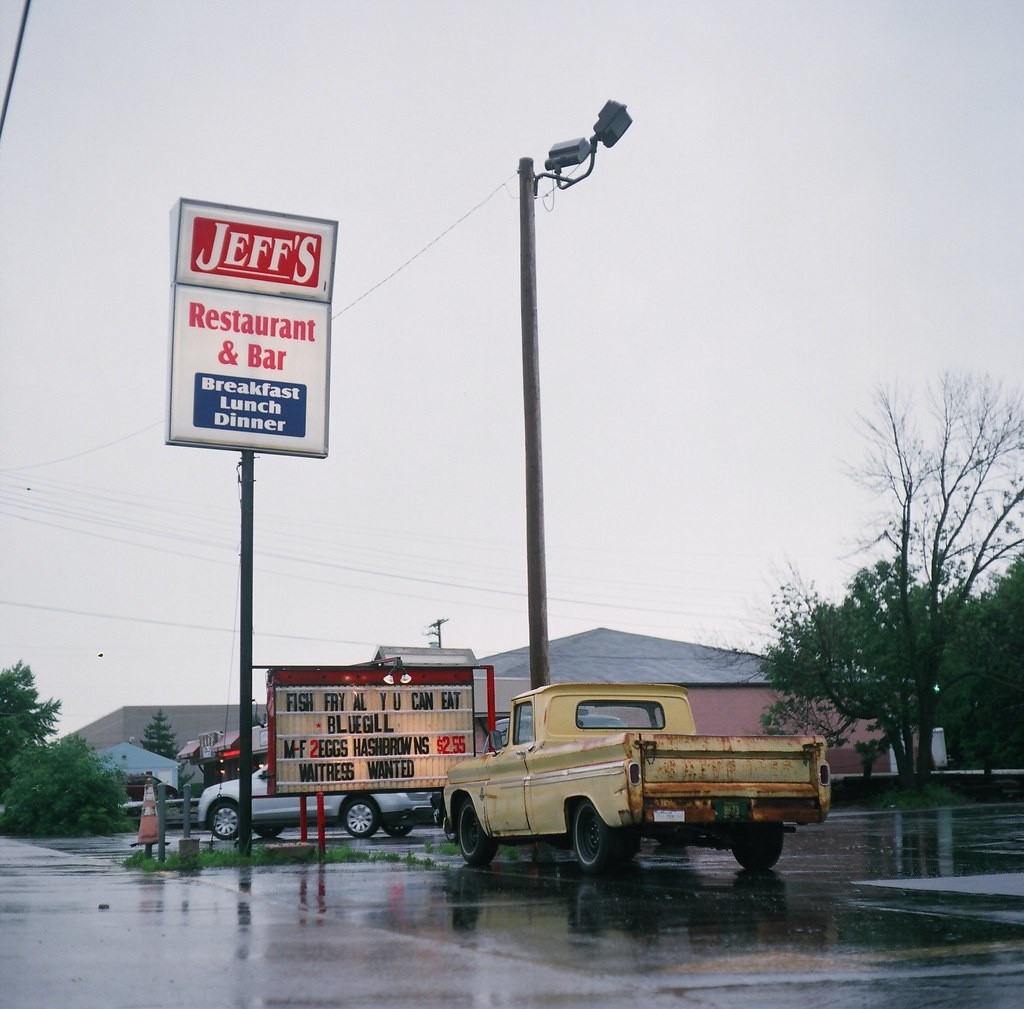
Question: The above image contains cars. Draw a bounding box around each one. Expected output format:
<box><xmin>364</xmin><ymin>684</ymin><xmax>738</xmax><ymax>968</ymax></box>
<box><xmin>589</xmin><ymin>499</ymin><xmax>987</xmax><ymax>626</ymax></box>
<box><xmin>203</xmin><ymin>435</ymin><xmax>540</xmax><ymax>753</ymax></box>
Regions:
<box><xmin>198</xmin><ymin>763</ymin><xmax>437</xmax><ymax>841</ymax></box>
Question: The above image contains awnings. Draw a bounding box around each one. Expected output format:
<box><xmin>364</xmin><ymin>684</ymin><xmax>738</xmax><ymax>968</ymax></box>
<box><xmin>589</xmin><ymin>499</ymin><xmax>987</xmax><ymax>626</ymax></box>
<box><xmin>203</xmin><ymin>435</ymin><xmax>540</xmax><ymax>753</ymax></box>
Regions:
<box><xmin>213</xmin><ymin>730</ymin><xmax>241</xmax><ymax>752</ymax></box>
<box><xmin>176</xmin><ymin>740</ymin><xmax>200</xmax><ymax>760</ymax></box>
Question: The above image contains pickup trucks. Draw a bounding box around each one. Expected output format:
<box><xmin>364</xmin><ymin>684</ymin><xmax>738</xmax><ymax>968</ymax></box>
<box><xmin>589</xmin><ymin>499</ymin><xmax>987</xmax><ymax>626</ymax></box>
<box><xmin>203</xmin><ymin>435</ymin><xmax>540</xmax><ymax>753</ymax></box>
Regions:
<box><xmin>440</xmin><ymin>681</ymin><xmax>833</xmax><ymax>880</ymax></box>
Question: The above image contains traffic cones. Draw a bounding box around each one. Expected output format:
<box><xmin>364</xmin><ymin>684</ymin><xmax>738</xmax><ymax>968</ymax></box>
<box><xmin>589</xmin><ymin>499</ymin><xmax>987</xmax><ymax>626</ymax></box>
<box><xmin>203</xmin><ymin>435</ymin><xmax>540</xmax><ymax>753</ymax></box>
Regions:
<box><xmin>128</xmin><ymin>777</ymin><xmax>171</xmax><ymax>847</ymax></box>
